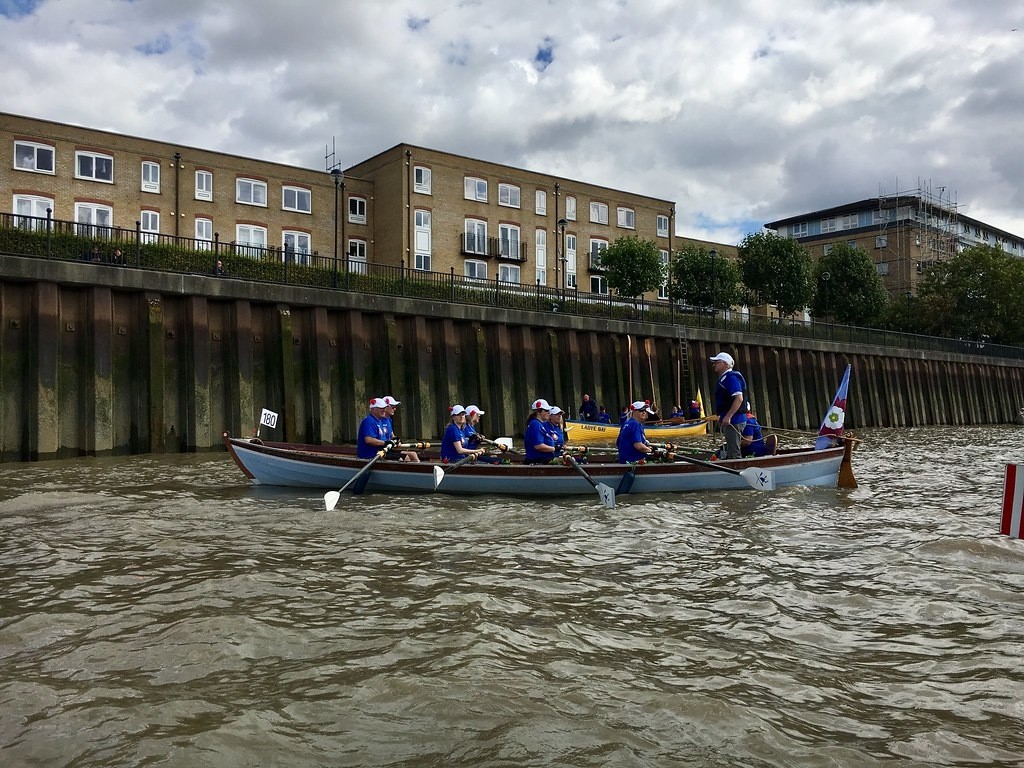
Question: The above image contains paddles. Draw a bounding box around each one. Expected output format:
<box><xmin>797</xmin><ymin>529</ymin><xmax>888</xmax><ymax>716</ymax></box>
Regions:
<box><xmin>432</xmin><ymin>449</ymin><xmax>486</xmax><ymax>491</ymax></box>
<box><xmin>677</xmin><ymin>359</ymin><xmax>680</xmax><ymax>408</ymax></box>
<box><xmin>564</xmin><ymin>446</ymin><xmax>619</xmax><ymax>452</ymax></box>
<box><xmin>627</xmin><ymin>335</ymin><xmax>633</xmax><ymax>417</ymax></box>
<box><xmin>472</xmin><ymin>434</ymin><xmax>518</xmax><ymax>454</ymax></box>
<box><xmin>323</xmin><ymin>439</ymin><xmax>397</xmax><ymax>511</ymax></box>
<box><xmin>654</xmin><ymin>450</ymin><xmax>777</xmax><ymax>491</ymax></box>
<box><xmin>644</xmin><ymin>338</ymin><xmax>657</xmax><ymax>407</ymax></box>
<box><xmin>560</xmin><ymin>448</ymin><xmax>616</xmax><ymax>510</ymax></box>
<box><xmin>650</xmin><ymin>441</ymin><xmax>727</xmax><ymax>453</ymax></box>
<box><xmin>397</xmin><ymin>436</ymin><xmax>514</xmax><ymax>449</ymax></box>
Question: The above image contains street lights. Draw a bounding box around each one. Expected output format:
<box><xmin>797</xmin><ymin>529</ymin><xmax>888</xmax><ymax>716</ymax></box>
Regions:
<box><xmin>822</xmin><ymin>271</ymin><xmax>831</xmax><ymax>340</ymax></box>
<box><xmin>558</xmin><ymin>218</ymin><xmax>569</xmax><ymax>310</ymax></box>
<box><xmin>905</xmin><ymin>291</ymin><xmax>912</xmax><ymax>348</ymax></box>
<box><xmin>708</xmin><ymin>250</ymin><xmax>718</xmax><ymax>328</ymax></box>
<box><xmin>329</xmin><ymin>169</ymin><xmax>345</xmax><ymax>287</ymax></box>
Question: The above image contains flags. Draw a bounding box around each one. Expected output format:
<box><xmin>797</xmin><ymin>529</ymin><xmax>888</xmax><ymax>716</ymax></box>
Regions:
<box><xmin>697</xmin><ymin>388</ymin><xmax>705</xmax><ymax>418</ymax></box>
<box><xmin>814</xmin><ymin>365</ymin><xmax>851</xmax><ymax>451</ymax></box>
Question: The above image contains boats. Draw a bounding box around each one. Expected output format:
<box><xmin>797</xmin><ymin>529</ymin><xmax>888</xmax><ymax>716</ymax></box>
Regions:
<box><xmin>562</xmin><ymin>400</ymin><xmax>710</xmax><ymax>441</ymax></box>
<box><xmin>221</xmin><ymin>431</ymin><xmax>862</xmax><ymax>490</ymax></box>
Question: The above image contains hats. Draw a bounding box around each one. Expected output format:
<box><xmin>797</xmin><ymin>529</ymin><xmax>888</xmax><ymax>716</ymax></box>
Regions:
<box><xmin>630</xmin><ymin>401</ymin><xmax>655</xmax><ymax>415</ymax></box>
<box><xmin>709</xmin><ymin>352</ymin><xmax>734</xmax><ymax>369</ymax></box>
<box><xmin>383</xmin><ymin>396</ymin><xmax>402</xmax><ymax>406</ymax></box>
<box><xmin>746</xmin><ymin>402</ymin><xmax>750</xmax><ymax>411</ymax></box>
<box><xmin>465</xmin><ymin>405</ymin><xmax>485</xmax><ymax>416</ymax></box>
<box><xmin>448</xmin><ymin>405</ymin><xmax>467</xmax><ymax>417</ymax></box>
<box><xmin>532</xmin><ymin>399</ymin><xmax>554</xmax><ymax>411</ymax></box>
<box><xmin>369</xmin><ymin>398</ymin><xmax>390</xmax><ymax>409</ymax></box>
<box><xmin>549</xmin><ymin>406</ymin><xmax>565</xmax><ymax>415</ymax></box>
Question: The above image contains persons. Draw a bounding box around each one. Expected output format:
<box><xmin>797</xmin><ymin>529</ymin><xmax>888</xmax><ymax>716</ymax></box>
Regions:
<box><xmin>357</xmin><ymin>398</ymin><xmax>410</xmax><ymax>462</ymax></box>
<box><xmin>741</xmin><ymin>400</ymin><xmax>765</xmax><ymax>458</ymax></box>
<box><xmin>523</xmin><ymin>398</ymin><xmax>566</xmax><ymax>465</ymax></box>
<box><xmin>619</xmin><ymin>392</ymin><xmax>699</xmax><ymax>427</ymax></box>
<box><xmin>578</xmin><ymin>394</ymin><xmax>598</xmax><ymax>423</ymax></box>
<box><xmin>543</xmin><ymin>406</ymin><xmax>586</xmax><ymax>465</ymax></box>
<box><xmin>465</xmin><ymin>406</ymin><xmax>501</xmax><ymax>464</ymax></box>
<box><xmin>597</xmin><ymin>405</ymin><xmax>611</xmax><ymax>424</ymax></box>
<box><xmin>382</xmin><ymin>396</ymin><xmax>420</xmax><ymax>462</ymax></box>
<box><xmin>616</xmin><ymin>400</ymin><xmax>669</xmax><ymax>465</ymax></box>
<box><xmin>441</xmin><ymin>405</ymin><xmax>484</xmax><ymax>464</ymax></box>
<box><xmin>710</xmin><ymin>352</ymin><xmax>746</xmax><ymax>459</ymax></box>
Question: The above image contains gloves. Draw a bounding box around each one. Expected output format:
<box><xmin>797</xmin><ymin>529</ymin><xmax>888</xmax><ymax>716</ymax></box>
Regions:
<box><xmin>648</xmin><ymin>445</ymin><xmax>658</xmax><ymax>454</ymax></box>
<box><xmin>554</xmin><ymin>444</ymin><xmax>564</xmax><ymax>452</ymax></box>
<box><xmin>383</xmin><ymin>436</ymin><xmax>401</xmax><ymax>450</ymax></box>
<box><xmin>468</xmin><ymin>433</ymin><xmax>486</xmax><ymax>443</ymax></box>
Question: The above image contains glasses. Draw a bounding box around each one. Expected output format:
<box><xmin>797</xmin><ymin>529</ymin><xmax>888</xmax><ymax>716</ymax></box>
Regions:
<box><xmin>540</xmin><ymin>408</ymin><xmax>549</xmax><ymax>412</ymax></box>
<box><xmin>390</xmin><ymin>406</ymin><xmax>397</xmax><ymax>409</ymax></box>
<box><xmin>713</xmin><ymin>361</ymin><xmax>726</xmax><ymax>366</ymax></box>
<box><xmin>638</xmin><ymin>405</ymin><xmax>649</xmax><ymax>412</ymax></box>
